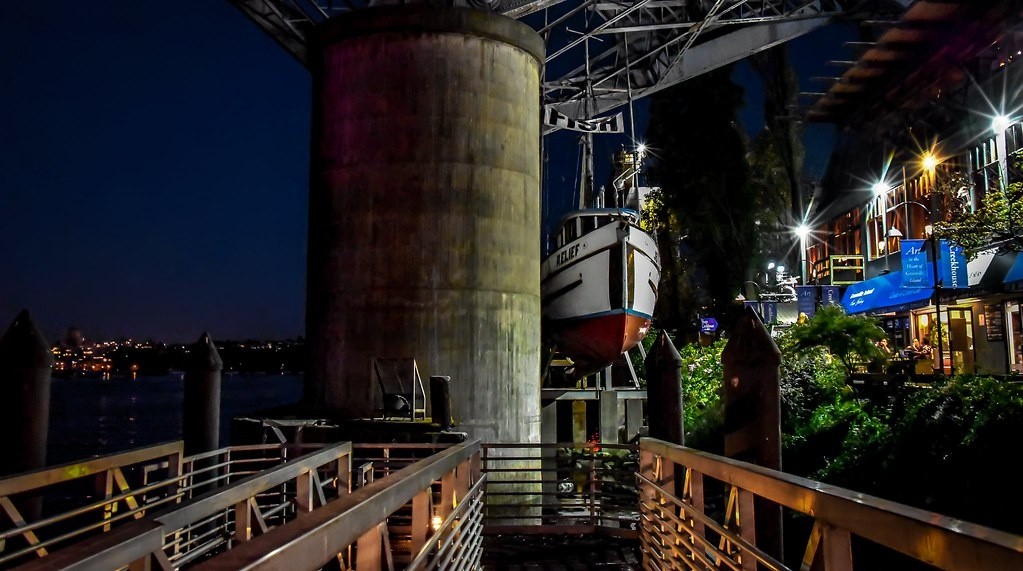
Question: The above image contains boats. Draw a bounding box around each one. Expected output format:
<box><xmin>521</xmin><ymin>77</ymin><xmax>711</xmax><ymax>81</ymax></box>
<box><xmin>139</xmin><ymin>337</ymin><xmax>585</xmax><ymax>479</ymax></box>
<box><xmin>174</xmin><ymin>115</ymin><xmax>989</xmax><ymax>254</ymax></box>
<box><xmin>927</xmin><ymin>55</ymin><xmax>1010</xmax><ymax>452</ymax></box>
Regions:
<box><xmin>541</xmin><ymin>126</ymin><xmax>663</xmax><ymax>409</ymax></box>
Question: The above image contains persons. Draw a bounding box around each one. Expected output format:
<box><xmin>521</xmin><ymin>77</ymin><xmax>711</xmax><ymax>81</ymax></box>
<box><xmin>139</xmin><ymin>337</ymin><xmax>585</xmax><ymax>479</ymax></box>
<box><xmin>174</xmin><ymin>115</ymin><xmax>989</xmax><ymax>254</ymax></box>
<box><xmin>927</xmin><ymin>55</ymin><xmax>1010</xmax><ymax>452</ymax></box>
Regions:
<box><xmin>876</xmin><ymin>338</ymin><xmax>928</xmax><ymax>361</ymax></box>
